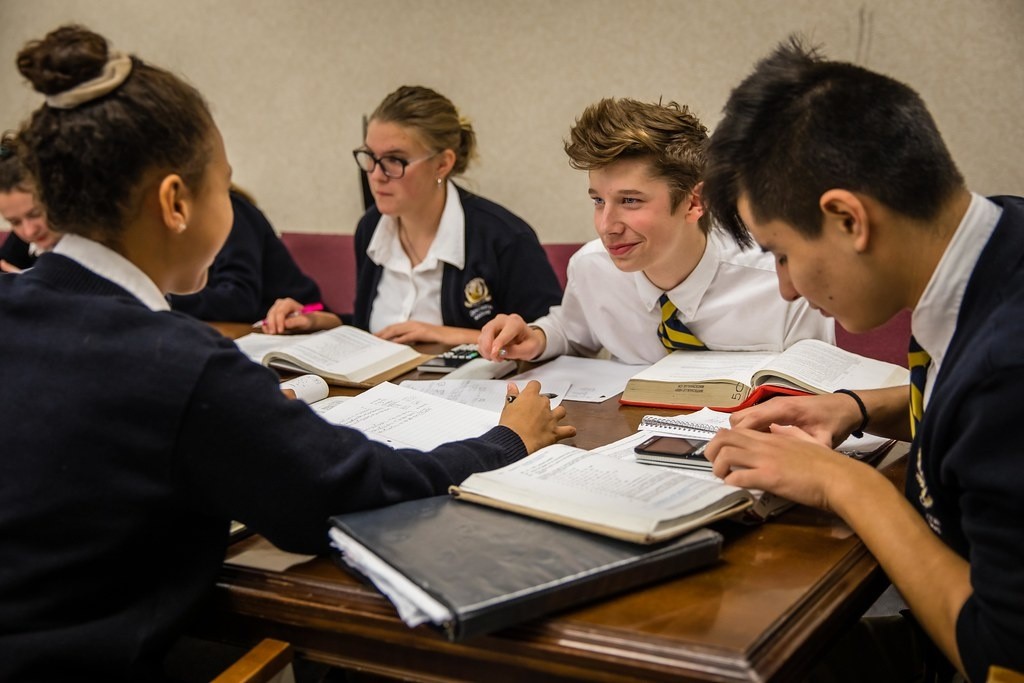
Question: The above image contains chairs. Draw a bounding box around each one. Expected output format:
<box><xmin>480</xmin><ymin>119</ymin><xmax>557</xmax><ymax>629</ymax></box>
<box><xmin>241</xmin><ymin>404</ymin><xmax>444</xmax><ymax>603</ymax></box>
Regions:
<box><xmin>834</xmin><ymin>306</ymin><xmax>912</xmax><ymax>369</ymax></box>
<box><xmin>282</xmin><ymin>233</ymin><xmax>356</xmax><ymax>314</ymax></box>
<box><xmin>540</xmin><ymin>244</ymin><xmax>586</xmax><ymax>290</ymax></box>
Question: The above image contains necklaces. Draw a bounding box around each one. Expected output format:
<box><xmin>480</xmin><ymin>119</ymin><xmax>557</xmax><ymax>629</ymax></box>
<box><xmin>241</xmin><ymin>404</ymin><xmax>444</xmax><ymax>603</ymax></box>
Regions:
<box><xmin>401</xmin><ymin>220</ymin><xmax>422</xmax><ymax>263</ymax></box>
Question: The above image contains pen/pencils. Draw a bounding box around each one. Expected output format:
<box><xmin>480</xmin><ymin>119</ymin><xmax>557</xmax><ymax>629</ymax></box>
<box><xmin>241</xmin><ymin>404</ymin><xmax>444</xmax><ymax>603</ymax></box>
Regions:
<box><xmin>498</xmin><ymin>348</ymin><xmax>507</xmax><ymax>356</ymax></box>
<box><xmin>506</xmin><ymin>393</ymin><xmax>558</xmax><ymax>403</ymax></box>
<box><xmin>252</xmin><ymin>301</ymin><xmax>323</xmax><ymax>328</ymax></box>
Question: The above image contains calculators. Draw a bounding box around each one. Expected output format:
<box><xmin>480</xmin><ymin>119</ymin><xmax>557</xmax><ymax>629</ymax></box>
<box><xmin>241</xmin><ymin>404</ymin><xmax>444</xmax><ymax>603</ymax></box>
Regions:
<box><xmin>416</xmin><ymin>344</ymin><xmax>480</xmax><ymax>374</ymax></box>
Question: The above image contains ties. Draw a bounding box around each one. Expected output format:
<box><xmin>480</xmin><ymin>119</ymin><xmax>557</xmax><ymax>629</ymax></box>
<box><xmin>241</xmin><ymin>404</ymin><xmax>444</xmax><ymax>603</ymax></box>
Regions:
<box><xmin>907</xmin><ymin>331</ymin><xmax>931</xmax><ymax>443</ymax></box>
<box><xmin>657</xmin><ymin>292</ymin><xmax>711</xmax><ymax>353</ymax></box>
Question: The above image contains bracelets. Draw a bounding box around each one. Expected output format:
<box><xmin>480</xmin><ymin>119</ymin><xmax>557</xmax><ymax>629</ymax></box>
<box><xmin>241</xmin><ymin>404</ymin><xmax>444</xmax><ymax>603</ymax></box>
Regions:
<box><xmin>832</xmin><ymin>389</ymin><xmax>868</xmax><ymax>439</ymax></box>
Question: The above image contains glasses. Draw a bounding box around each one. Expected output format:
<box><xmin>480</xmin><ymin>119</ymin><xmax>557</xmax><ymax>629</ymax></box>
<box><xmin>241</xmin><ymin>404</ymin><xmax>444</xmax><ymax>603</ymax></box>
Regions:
<box><xmin>353</xmin><ymin>147</ymin><xmax>443</xmax><ymax>180</ymax></box>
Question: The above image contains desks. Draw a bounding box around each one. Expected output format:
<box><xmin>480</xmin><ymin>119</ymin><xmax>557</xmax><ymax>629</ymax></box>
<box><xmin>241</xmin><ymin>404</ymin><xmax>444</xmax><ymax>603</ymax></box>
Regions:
<box><xmin>205</xmin><ymin>321</ymin><xmax>909</xmax><ymax>683</ymax></box>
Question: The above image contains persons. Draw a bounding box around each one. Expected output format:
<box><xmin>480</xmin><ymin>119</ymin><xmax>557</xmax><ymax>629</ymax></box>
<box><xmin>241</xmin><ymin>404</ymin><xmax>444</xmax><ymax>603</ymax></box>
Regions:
<box><xmin>0</xmin><ymin>23</ymin><xmax>576</xmax><ymax>683</ymax></box>
<box><xmin>165</xmin><ymin>185</ymin><xmax>326</xmax><ymax>325</ymax></box>
<box><xmin>476</xmin><ymin>94</ymin><xmax>836</xmax><ymax>363</ymax></box>
<box><xmin>699</xmin><ymin>42</ymin><xmax>1024</xmax><ymax>683</ymax></box>
<box><xmin>263</xmin><ymin>85</ymin><xmax>566</xmax><ymax>344</ymax></box>
<box><xmin>0</xmin><ymin>137</ymin><xmax>63</xmax><ymax>274</ymax></box>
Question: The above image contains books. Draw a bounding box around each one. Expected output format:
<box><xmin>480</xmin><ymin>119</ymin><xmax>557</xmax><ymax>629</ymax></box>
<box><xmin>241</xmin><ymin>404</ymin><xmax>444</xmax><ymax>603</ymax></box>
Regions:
<box><xmin>229</xmin><ymin>326</ymin><xmax>911</xmax><ymax>644</ymax></box>
